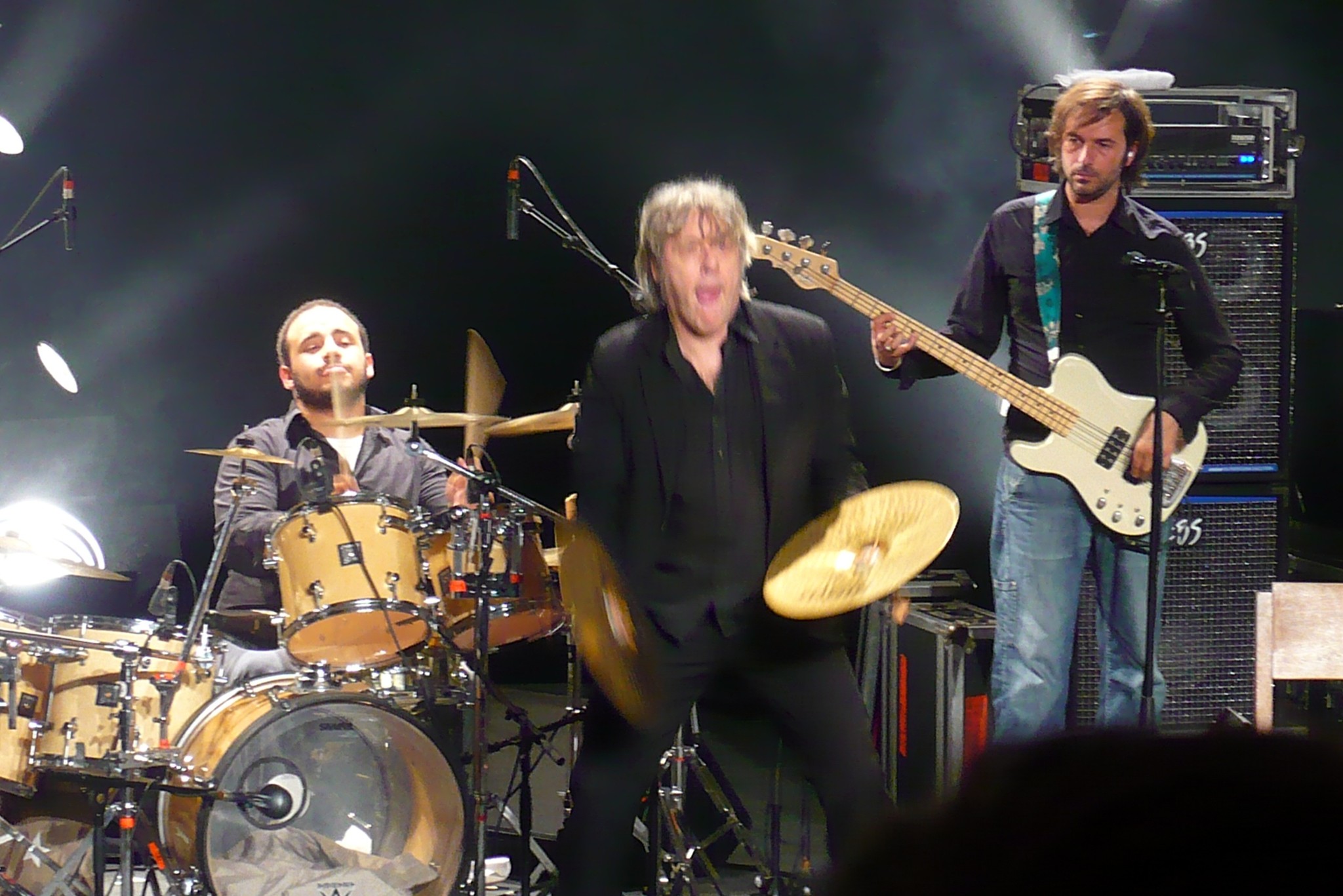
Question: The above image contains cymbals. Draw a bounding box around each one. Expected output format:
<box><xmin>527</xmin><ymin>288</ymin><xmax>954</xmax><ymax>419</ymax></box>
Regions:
<box><xmin>761</xmin><ymin>478</ymin><xmax>962</xmax><ymax>622</ymax></box>
<box><xmin>1</xmin><ymin>547</ymin><xmax>133</xmax><ymax>582</ymax></box>
<box><xmin>482</xmin><ymin>402</ymin><xmax>581</xmax><ymax>438</ymax></box>
<box><xmin>181</xmin><ymin>445</ymin><xmax>297</xmax><ymax>467</ymax></box>
<box><xmin>553</xmin><ymin>520</ymin><xmax>665</xmax><ymax>736</ymax></box>
<box><xmin>314</xmin><ymin>405</ymin><xmax>511</xmax><ymax>432</ymax></box>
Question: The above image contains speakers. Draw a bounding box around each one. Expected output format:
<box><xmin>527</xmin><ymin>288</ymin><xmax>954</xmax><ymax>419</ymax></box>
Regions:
<box><xmin>1069</xmin><ymin>482</ymin><xmax>1289</xmax><ymax>733</ymax></box>
<box><xmin>1127</xmin><ymin>197</ymin><xmax>1297</xmax><ymax>482</ymax></box>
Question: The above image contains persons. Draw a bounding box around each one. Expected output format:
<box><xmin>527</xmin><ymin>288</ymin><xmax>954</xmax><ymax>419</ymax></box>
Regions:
<box><xmin>871</xmin><ymin>79</ymin><xmax>1244</xmax><ymax>740</ymax></box>
<box><xmin>560</xmin><ymin>179</ymin><xmax>896</xmax><ymax>896</ymax></box>
<box><xmin>213</xmin><ymin>297</ymin><xmax>495</xmax><ymax>896</ymax></box>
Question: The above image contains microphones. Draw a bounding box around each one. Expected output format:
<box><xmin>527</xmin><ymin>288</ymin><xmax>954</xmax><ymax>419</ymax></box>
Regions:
<box><xmin>219</xmin><ymin>784</ymin><xmax>293</xmax><ymax>819</ymax></box>
<box><xmin>62</xmin><ymin>169</ymin><xmax>75</xmax><ymax>250</ymax></box>
<box><xmin>311</xmin><ymin>439</ymin><xmax>335</xmax><ymax>492</ymax></box>
<box><xmin>468</xmin><ymin>445</ymin><xmax>484</xmax><ymax>504</ymax></box>
<box><xmin>507</xmin><ymin>160</ymin><xmax>520</xmax><ymax>240</ymax></box>
<box><xmin>148</xmin><ymin>563</ymin><xmax>176</xmax><ymax>616</ymax></box>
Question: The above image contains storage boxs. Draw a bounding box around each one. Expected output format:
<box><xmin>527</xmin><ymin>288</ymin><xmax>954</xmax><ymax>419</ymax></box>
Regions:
<box><xmin>881</xmin><ymin>599</ymin><xmax>999</xmax><ymax>820</ymax></box>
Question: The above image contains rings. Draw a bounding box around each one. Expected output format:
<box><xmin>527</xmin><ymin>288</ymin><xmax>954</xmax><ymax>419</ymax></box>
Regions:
<box><xmin>886</xmin><ymin>346</ymin><xmax>891</xmax><ymax>351</ymax></box>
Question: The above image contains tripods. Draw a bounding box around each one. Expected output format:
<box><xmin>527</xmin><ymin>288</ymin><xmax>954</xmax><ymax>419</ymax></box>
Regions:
<box><xmin>650</xmin><ymin>724</ymin><xmax>784</xmax><ymax>896</ymax></box>
<box><xmin>0</xmin><ymin>628</ymin><xmax>217</xmax><ymax>896</ymax></box>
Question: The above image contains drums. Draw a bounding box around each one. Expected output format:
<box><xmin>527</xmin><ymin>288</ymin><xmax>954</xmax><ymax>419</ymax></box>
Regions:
<box><xmin>421</xmin><ymin>498</ymin><xmax>568</xmax><ymax>654</ymax></box>
<box><xmin>266</xmin><ymin>489</ymin><xmax>437</xmax><ymax>673</ymax></box>
<box><xmin>151</xmin><ymin>671</ymin><xmax>479</xmax><ymax>896</ymax></box>
<box><xmin>341</xmin><ymin>645</ymin><xmax>468</xmax><ymax>706</ymax></box>
<box><xmin>0</xmin><ymin>604</ymin><xmax>53</xmax><ymax>801</ymax></box>
<box><xmin>31</xmin><ymin>609</ymin><xmax>221</xmax><ymax>784</ymax></box>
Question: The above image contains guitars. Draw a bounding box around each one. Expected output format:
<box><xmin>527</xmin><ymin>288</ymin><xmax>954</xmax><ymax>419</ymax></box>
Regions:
<box><xmin>750</xmin><ymin>218</ymin><xmax>1210</xmax><ymax>545</ymax></box>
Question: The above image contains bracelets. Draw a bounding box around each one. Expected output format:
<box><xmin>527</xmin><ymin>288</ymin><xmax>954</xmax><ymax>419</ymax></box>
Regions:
<box><xmin>875</xmin><ymin>358</ymin><xmax>901</xmax><ymax>372</ymax></box>
